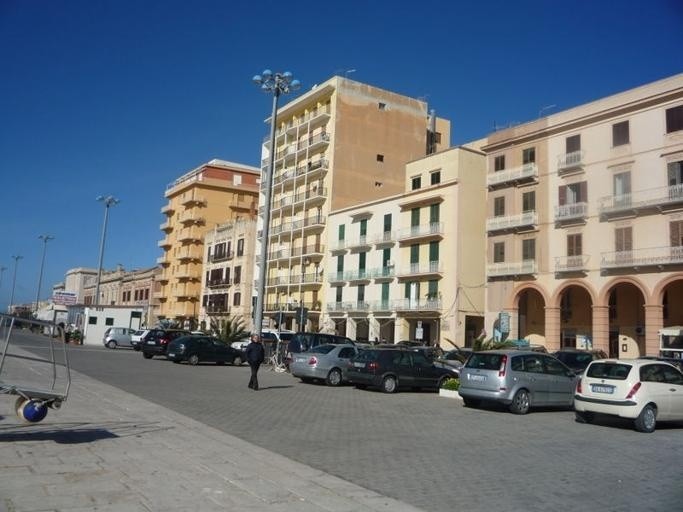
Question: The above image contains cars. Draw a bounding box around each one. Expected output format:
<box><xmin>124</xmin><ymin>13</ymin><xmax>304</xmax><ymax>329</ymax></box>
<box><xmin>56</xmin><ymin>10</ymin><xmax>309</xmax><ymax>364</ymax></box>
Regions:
<box><xmin>638</xmin><ymin>355</ymin><xmax>683</xmax><ymax>372</ymax></box>
<box><xmin>432</xmin><ymin>347</ymin><xmax>472</xmax><ymax>375</ymax></box>
<box><xmin>458</xmin><ymin>349</ymin><xmax>583</xmax><ymax>415</ymax></box>
<box><xmin>103</xmin><ymin>327</ymin><xmax>136</xmax><ymax>350</ymax></box>
<box><xmin>573</xmin><ymin>358</ymin><xmax>683</xmax><ymax>433</ymax></box>
<box><xmin>230</xmin><ymin>329</ymin><xmax>433</xmax><ymax>361</ymax></box>
<box><xmin>553</xmin><ymin>347</ymin><xmax>607</xmax><ymax>376</ymax></box>
<box><xmin>288</xmin><ymin>343</ymin><xmax>367</xmax><ymax>386</ymax></box>
<box><xmin>347</xmin><ymin>347</ymin><xmax>460</xmax><ymax>394</ymax></box>
<box><xmin>166</xmin><ymin>334</ymin><xmax>247</xmax><ymax>366</ymax></box>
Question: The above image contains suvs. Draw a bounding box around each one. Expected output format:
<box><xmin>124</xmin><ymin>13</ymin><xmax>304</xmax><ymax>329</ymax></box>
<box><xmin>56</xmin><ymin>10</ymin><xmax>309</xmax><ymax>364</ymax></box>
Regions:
<box><xmin>130</xmin><ymin>330</ymin><xmax>152</xmax><ymax>351</ymax></box>
<box><xmin>140</xmin><ymin>329</ymin><xmax>192</xmax><ymax>359</ymax></box>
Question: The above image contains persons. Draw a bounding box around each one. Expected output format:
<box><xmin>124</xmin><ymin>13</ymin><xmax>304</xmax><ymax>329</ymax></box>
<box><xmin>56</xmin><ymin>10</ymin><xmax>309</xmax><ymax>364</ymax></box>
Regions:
<box><xmin>373</xmin><ymin>337</ymin><xmax>379</xmax><ymax>344</ymax></box>
<box><xmin>381</xmin><ymin>337</ymin><xmax>386</xmax><ymax>344</ymax></box>
<box><xmin>245</xmin><ymin>333</ymin><xmax>264</xmax><ymax>391</ymax></box>
<box><xmin>353</xmin><ymin>336</ymin><xmax>361</xmax><ymax>343</ymax></box>
<box><xmin>432</xmin><ymin>339</ymin><xmax>439</xmax><ymax>348</ymax></box>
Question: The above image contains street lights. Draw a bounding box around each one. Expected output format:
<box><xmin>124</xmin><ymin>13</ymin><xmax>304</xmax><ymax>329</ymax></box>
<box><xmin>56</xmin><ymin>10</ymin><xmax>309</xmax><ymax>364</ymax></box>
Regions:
<box><xmin>33</xmin><ymin>234</ymin><xmax>54</xmax><ymax>312</ymax></box>
<box><xmin>0</xmin><ymin>253</ymin><xmax>24</xmax><ymax>316</ymax></box>
<box><xmin>93</xmin><ymin>195</ymin><xmax>120</xmax><ymax>305</ymax></box>
<box><xmin>250</xmin><ymin>68</ymin><xmax>301</xmax><ymax>345</ymax></box>
